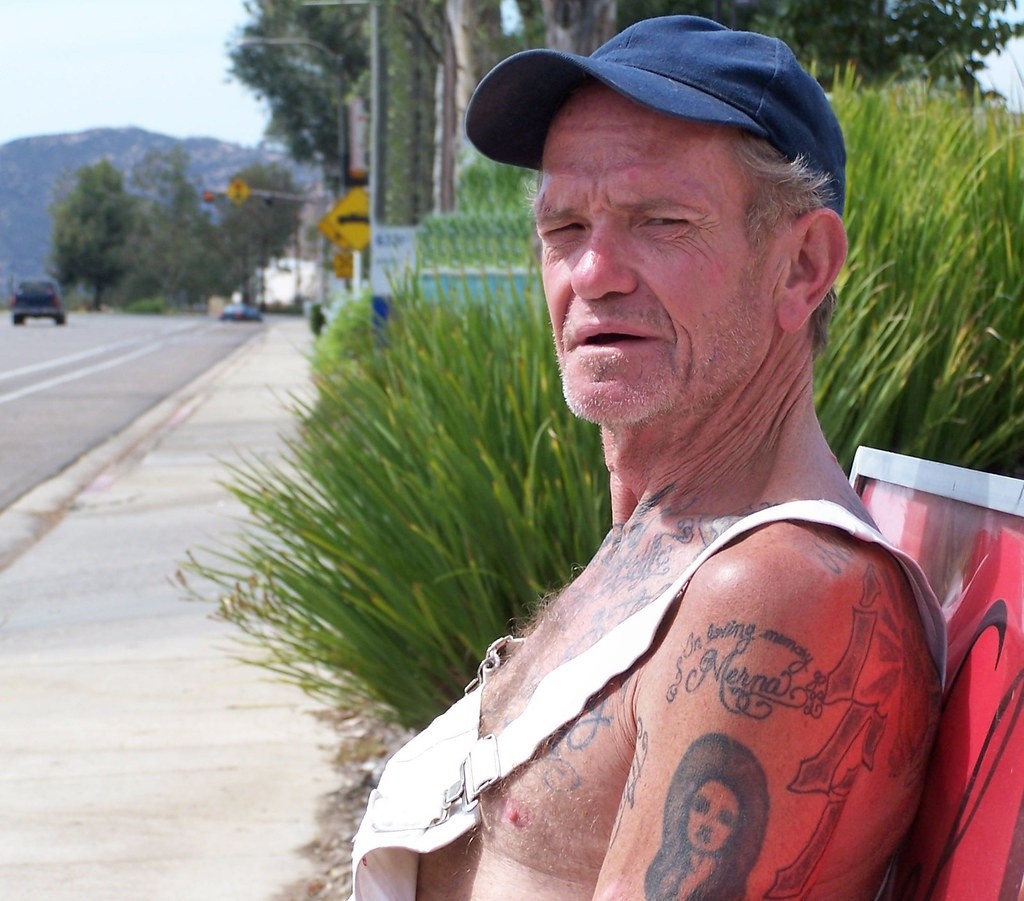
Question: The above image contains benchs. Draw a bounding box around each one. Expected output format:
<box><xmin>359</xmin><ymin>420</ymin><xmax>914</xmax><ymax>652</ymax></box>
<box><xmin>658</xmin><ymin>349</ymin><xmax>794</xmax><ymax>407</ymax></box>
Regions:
<box><xmin>800</xmin><ymin>446</ymin><xmax>1024</xmax><ymax>901</ymax></box>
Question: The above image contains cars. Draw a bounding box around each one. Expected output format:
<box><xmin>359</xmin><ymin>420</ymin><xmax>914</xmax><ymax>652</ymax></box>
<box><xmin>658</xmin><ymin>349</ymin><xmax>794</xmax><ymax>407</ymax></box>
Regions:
<box><xmin>12</xmin><ymin>279</ymin><xmax>67</xmax><ymax>325</ymax></box>
<box><xmin>218</xmin><ymin>303</ymin><xmax>263</xmax><ymax>321</ymax></box>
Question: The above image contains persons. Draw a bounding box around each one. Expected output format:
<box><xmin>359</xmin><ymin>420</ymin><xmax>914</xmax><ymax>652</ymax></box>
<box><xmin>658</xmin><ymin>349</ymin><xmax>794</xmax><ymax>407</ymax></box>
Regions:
<box><xmin>353</xmin><ymin>15</ymin><xmax>956</xmax><ymax>901</ymax></box>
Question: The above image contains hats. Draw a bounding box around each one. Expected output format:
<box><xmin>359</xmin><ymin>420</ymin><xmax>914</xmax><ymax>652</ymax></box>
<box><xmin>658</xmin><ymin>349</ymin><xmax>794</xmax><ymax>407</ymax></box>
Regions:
<box><xmin>465</xmin><ymin>13</ymin><xmax>845</xmax><ymax>219</ymax></box>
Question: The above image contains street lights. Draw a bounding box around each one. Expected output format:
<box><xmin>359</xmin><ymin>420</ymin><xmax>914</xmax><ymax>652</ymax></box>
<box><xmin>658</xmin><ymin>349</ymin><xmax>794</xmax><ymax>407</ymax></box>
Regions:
<box><xmin>235</xmin><ymin>37</ymin><xmax>344</xmax><ymax>132</ymax></box>
